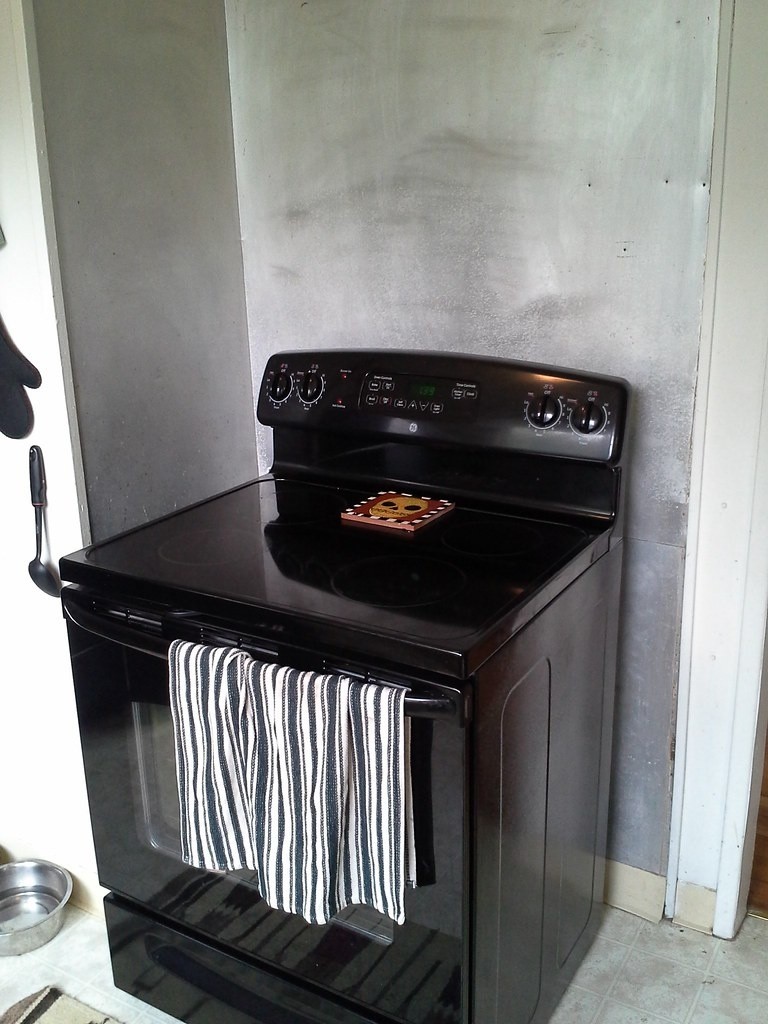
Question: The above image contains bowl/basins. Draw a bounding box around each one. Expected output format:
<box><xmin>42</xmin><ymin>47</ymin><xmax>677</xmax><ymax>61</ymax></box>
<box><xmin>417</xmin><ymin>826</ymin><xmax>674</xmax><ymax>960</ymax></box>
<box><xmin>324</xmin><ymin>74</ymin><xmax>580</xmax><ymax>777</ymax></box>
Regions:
<box><xmin>0</xmin><ymin>859</ymin><xmax>74</xmax><ymax>956</ymax></box>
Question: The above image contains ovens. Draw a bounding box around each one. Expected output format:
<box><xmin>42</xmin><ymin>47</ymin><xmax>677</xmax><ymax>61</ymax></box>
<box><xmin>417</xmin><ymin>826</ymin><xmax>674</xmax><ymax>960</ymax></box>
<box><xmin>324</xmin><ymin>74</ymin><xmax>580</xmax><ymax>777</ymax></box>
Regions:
<box><xmin>60</xmin><ymin>345</ymin><xmax>634</xmax><ymax>1024</ymax></box>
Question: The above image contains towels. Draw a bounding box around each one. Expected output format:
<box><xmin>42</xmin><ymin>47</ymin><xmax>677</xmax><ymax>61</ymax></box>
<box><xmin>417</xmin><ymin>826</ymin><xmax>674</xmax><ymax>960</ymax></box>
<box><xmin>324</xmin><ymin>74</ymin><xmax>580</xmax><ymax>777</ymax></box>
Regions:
<box><xmin>162</xmin><ymin>634</ymin><xmax>422</xmax><ymax>926</ymax></box>
